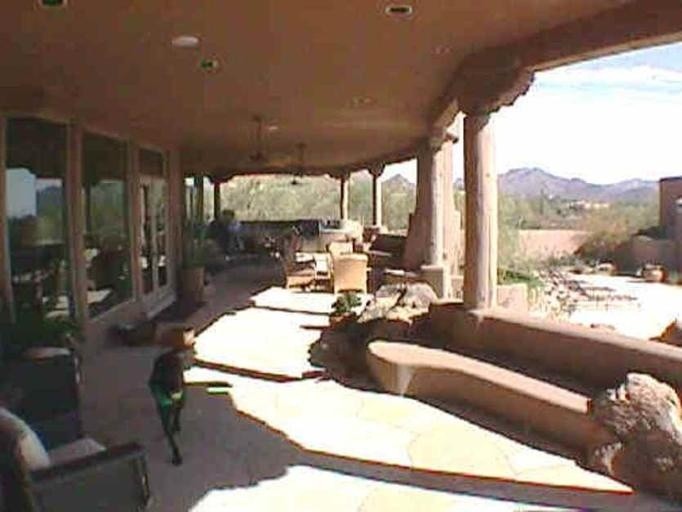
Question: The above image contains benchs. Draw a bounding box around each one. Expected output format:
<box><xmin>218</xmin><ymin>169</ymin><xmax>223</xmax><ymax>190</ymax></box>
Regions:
<box><xmin>364</xmin><ymin>298</ymin><xmax>681</xmax><ymax>499</ymax></box>
<box><xmin>358</xmin><ymin>262</ymin><xmax>443</xmax><ymax>323</ymax></box>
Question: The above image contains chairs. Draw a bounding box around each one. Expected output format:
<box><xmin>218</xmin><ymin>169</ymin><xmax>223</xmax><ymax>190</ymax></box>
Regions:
<box><xmin>15</xmin><ymin>344</ymin><xmax>83</xmax><ymax>384</ymax></box>
<box><xmin>367</xmin><ymin>212</ymin><xmax>432</xmax><ymax>293</ymax></box>
<box><xmin>326</xmin><ymin>238</ymin><xmax>356</xmax><ymax>270</ymax></box>
<box><xmin>330</xmin><ymin>253</ymin><xmax>373</xmax><ymax>291</ymax></box>
<box><xmin>276</xmin><ymin>246</ymin><xmax>317</xmax><ymax>292</ymax></box>
<box><xmin>0</xmin><ymin>407</ymin><xmax>153</xmax><ymax>511</ymax></box>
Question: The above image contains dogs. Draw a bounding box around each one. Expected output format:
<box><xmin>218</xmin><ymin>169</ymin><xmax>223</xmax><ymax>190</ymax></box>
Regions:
<box><xmin>148</xmin><ymin>347</ymin><xmax>186</xmax><ymax>466</ymax></box>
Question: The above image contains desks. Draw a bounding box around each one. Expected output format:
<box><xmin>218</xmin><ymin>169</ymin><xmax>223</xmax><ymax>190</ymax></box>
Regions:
<box><xmin>0</xmin><ymin>353</ymin><xmax>83</xmax><ymax>438</ymax></box>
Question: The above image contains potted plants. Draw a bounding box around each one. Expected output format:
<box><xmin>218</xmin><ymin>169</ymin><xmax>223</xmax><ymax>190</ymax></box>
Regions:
<box><xmin>174</xmin><ymin>216</ymin><xmax>210</xmax><ymax>306</ymax></box>
<box><xmin>327</xmin><ymin>290</ymin><xmax>363</xmax><ymax>325</ymax></box>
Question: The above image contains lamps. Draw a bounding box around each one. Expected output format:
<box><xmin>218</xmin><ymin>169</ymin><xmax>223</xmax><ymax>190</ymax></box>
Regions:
<box><xmin>18</xmin><ymin>213</ymin><xmax>66</xmax><ymax>311</ymax></box>
